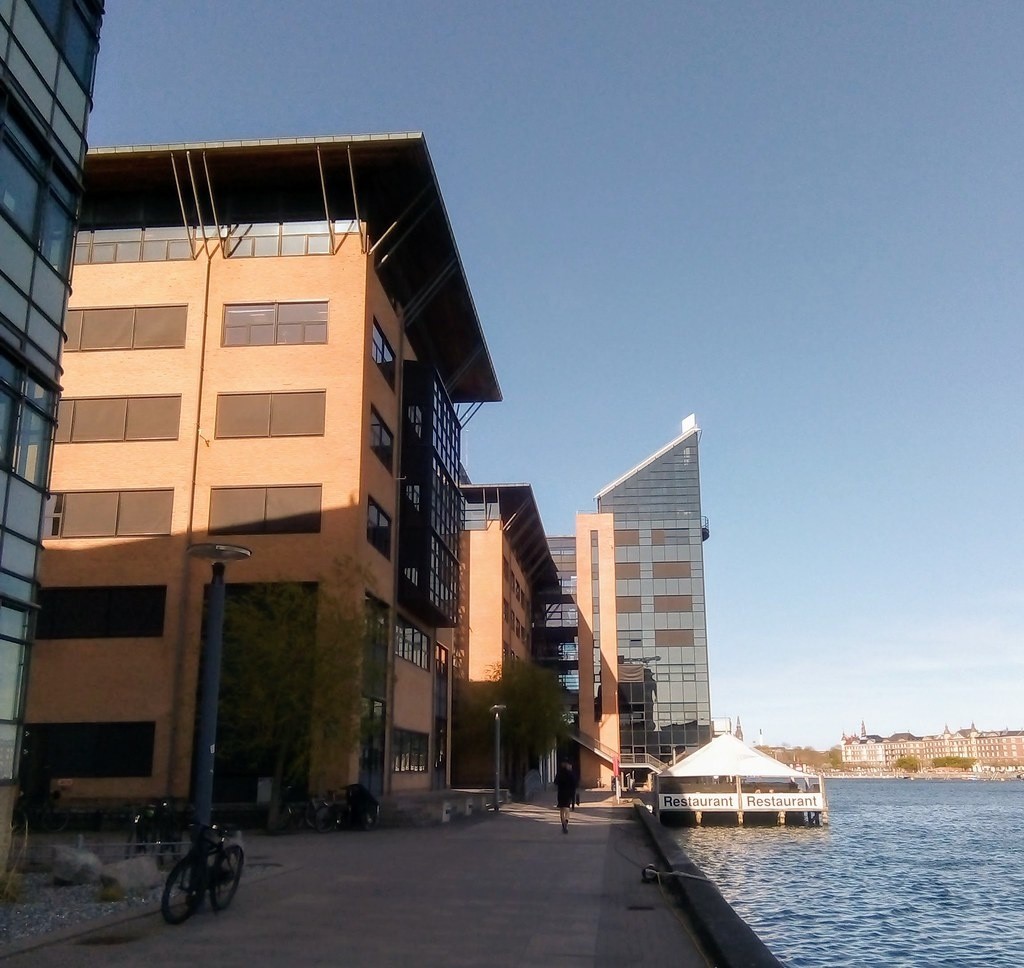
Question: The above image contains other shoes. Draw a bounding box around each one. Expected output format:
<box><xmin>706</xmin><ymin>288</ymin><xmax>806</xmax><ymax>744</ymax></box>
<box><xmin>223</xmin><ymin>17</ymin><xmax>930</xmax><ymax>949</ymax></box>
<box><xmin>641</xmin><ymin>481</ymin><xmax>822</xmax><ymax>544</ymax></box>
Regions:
<box><xmin>563</xmin><ymin>826</ymin><xmax>568</xmax><ymax>832</ymax></box>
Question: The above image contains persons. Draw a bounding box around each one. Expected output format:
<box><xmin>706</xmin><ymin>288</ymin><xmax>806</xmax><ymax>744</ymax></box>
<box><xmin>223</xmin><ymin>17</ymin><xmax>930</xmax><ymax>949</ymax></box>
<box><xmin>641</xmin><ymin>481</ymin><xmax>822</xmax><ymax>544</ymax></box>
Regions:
<box><xmin>554</xmin><ymin>756</ymin><xmax>578</xmax><ymax>834</ymax></box>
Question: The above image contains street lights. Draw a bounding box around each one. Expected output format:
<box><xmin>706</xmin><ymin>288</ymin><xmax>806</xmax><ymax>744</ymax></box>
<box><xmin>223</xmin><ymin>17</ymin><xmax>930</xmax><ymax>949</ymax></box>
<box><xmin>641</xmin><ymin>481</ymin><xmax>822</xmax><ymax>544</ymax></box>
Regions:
<box><xmin>184</xmin><ymin>541</ymin><xmax>251</xmax><ymax>828</ymax></box>
<box><xmin>489</xmin><ymin>703</ymin><xmax>507</xmax><ymax>811</ymax></box>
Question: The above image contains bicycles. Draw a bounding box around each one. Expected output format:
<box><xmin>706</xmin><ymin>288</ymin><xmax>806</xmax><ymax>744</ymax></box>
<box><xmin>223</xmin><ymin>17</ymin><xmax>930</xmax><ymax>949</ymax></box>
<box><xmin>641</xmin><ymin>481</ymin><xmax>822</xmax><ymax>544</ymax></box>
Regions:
<box><xmin>160</xmin><ymin>819</ymin><xmax>246</xmax><ymax>926</ymax></box>
<box><xmin>263</xmin><ymin>783</ymin><xmax>381</xmax><ymax>835</ymax></box>
